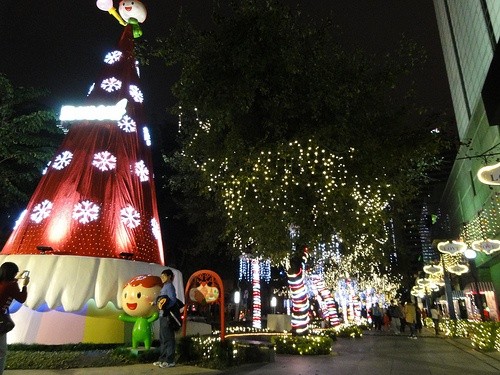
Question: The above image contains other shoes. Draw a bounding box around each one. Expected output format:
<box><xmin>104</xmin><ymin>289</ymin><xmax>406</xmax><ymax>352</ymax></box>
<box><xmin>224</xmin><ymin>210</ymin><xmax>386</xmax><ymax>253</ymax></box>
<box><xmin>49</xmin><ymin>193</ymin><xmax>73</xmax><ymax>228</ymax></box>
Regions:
<box><xmin>408</xmin><ymin>335</ymin><xmax>418</xmax><ymax>340</ymax></box>
<box><xmin>153</xmin><ymin>360</ymin><xmax>175</xmax><ymax>368</ymax></box>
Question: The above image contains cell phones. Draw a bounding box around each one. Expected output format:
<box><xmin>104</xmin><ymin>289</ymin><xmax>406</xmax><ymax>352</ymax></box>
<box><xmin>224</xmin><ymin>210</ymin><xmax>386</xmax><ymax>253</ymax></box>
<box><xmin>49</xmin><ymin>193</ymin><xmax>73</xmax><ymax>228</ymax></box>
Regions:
<box><xmin>24</xmin><ymin>271</ymin><xmax>29</xmax><ymax>278</ymax></box>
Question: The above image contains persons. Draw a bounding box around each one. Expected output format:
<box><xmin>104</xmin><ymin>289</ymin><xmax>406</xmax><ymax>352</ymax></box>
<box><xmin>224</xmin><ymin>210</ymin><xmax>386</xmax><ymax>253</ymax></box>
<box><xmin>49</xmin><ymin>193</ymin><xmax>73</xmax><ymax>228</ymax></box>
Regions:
<box><xmin>367</xmin><ymin>298</ymin><xmax>441</xmax><ymax>341</ymax></box>
<box><xmin>153</xmin><ymin>268</ymin><xmax>181</xmax><ymax>368</ymax></box>
<box><xmin>0</xmin><ymin>262</ymin><xmax>29</xmax><ymax>375</ymax></box>
